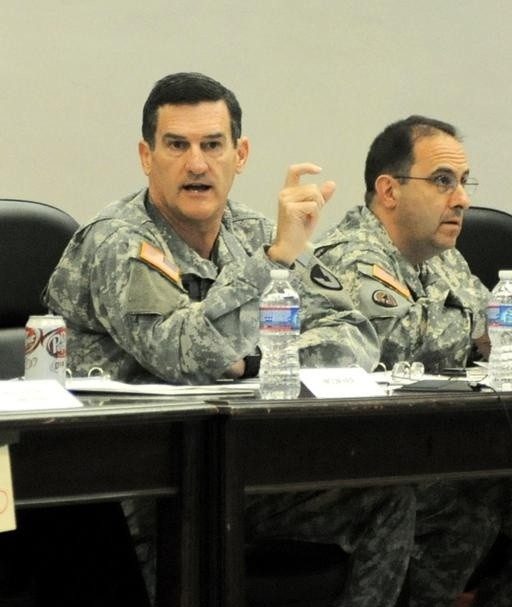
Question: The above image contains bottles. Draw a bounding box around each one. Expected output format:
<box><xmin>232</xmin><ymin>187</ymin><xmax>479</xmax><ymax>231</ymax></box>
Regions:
<box><xmin>24</xmin><ymin>313</ymin><xmax>67</xmax><ymax>391</ymax></box>
<box><xmin>485</xmin><ymin>270</ymin><xmax>512</xmax><ymax>394</ymax></box>
<box><xmin>257</xmin><ymin>269</ymin><xmax>302</xmax><ymax>402</ymax></box>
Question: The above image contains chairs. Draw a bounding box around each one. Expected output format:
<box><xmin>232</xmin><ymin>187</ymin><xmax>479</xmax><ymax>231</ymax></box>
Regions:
<box><xmin>0</xmin><ymin>198</ymin><xmax>82</xmax><ymax>329</ymax></box>
<box><xmin>456</xmin><ymin>207</ymin><xmax>512</xmax><ymax>292</ymax></box>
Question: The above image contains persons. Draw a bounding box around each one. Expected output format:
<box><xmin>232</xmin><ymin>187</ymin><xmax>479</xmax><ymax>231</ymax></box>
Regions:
<box><xmin>312</xmin><ymin>116</ymin><xmax>512</xmax><ymax>607</ymax></box>
<box><xmin>40</xmin><ymin>72</ymin><xmax>417</xmax><ymax>607</ymax></box>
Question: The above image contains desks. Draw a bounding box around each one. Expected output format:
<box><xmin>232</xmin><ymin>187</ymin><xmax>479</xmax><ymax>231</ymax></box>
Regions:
<box><xmin>0</xmin><ymin>371</ymin><xmax>512</xmax><ymax>607</ymax></box>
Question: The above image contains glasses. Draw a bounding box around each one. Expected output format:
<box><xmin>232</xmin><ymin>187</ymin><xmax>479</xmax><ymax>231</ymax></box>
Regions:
<box><xmin>392</xmin><ymin>172</ymin><xmax>478</xmax><ymax>194</ymax></box>
<box><xmin>390</xmin><ymin>359</ymin><xmax>489</xmax><ymax>386</ymax></box>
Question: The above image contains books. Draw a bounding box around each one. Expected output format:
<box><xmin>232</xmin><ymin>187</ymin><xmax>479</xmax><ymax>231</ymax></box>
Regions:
<box><xmin>440</xmin><ymin>366</ymin><xmax>487</xmax><ymax>380</ymax></box>
